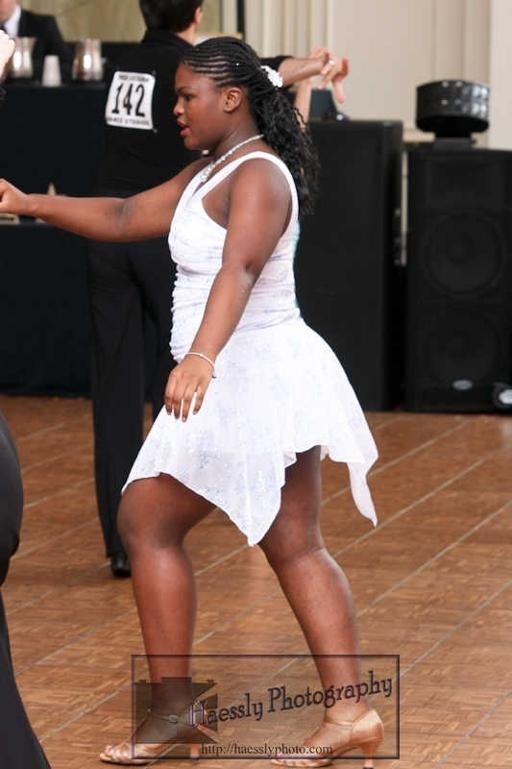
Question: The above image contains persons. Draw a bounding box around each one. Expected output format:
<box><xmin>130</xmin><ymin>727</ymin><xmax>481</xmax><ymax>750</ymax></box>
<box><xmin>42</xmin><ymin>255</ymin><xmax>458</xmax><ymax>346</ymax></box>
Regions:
<box><xmin>1</xmin><ymin>32</ymin><xmax>385</xmax><ymax>769</ymax></box>
<box><xmin>0</xmin><ymin>2</ymin><xmax>76</xmax><ymax>78</ymax></box>
<box><xmin>87</xmin><ymin>0</ymin><xmax>331</xmax><ymax>578</ymax></box>
<box><xmin>1</xmin><ymin>416</ymin><xmax>59</xmax><ymax>769</ymax></box>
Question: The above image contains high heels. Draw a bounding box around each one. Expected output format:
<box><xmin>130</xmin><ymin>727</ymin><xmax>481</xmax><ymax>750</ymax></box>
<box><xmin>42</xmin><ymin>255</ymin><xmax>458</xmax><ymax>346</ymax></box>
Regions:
<box><xmin>99</xmin><ymin>701</ymin><xmax>209</xmax><ymax>766</ymax></box>
<box><xmin>269</xmin><ymin>707</ymin><xmax>385</xmax><ymax>769</ymax></box>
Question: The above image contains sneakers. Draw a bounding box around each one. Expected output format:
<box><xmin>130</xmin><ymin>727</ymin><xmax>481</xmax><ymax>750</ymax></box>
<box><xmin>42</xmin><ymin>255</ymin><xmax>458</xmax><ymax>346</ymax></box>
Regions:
<box><xmin>111</xmin><ymin>549</ymin><xmax>131</xmax><ymax>577</ymax></box>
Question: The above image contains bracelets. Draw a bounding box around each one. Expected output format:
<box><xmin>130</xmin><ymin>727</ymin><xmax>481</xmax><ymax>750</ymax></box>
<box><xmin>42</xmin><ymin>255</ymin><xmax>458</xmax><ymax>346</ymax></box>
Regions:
<box><xmin>181</xmin><ymin>349</ymin><xmax>218</xmax><ymax>374</ymax></box>
